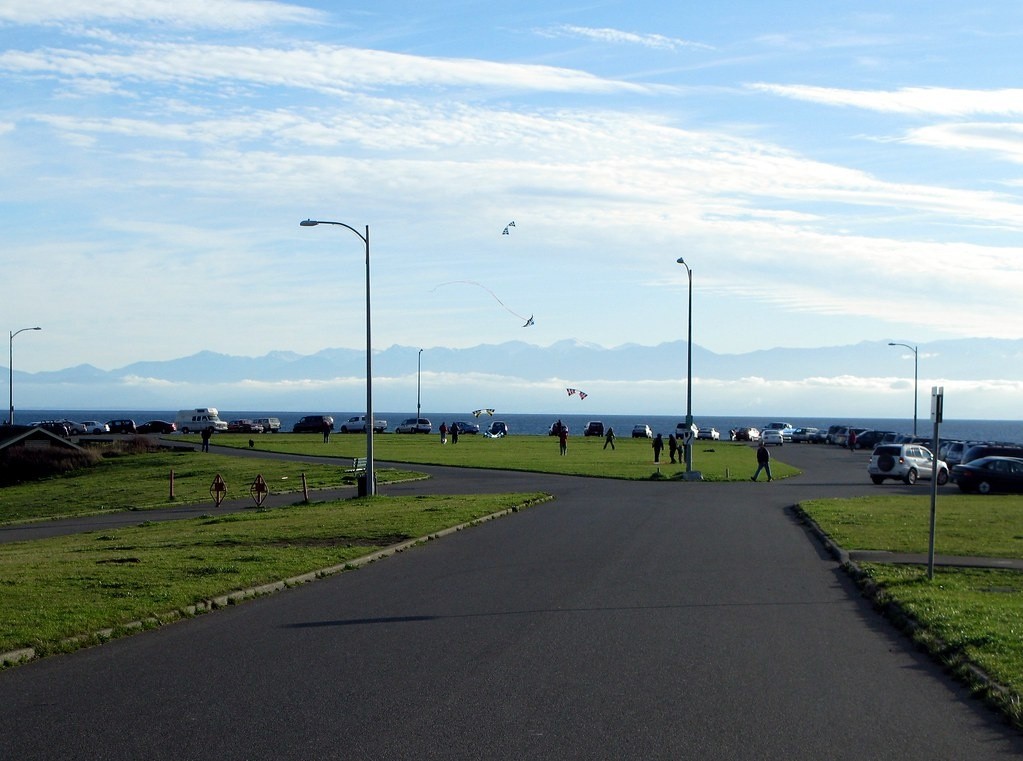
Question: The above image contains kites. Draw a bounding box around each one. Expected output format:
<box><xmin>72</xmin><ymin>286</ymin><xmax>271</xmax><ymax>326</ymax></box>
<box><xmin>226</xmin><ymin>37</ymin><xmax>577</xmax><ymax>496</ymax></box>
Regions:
<box><xmin>520</xmin><ymin>313</ymin><xmax>535</xmax><ymax>328</ymax></box>
<box><xmin>567</xmin><ymin>388</ymin><xmax>588</xmax><ymax>400</ymax></box>
<box><xmin>501</xmin><ymin>220</ymin><xmax>516</xmax><ymax>235</ymax></box>
<box><xmin>472</xmin><ymin>409</ymin><xmax>496</xmax><ymax>418</ymax></box>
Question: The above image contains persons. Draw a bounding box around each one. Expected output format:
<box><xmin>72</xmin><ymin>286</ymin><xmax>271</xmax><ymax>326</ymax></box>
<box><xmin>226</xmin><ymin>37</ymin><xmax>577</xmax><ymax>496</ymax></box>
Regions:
<box><xmin>653</xmin><ymin>433</ymin><xmax>683</xmax><ymax>464</ymax></box>
<box><xmin>850</xmin><ymin>430</ymin><xmax>856</xmax><ymax>452</ymax></box>
<box><xmin>202</xmin><ymin>426</ymin><xmax>211</xmax><ymax>453</ymax></box>
<box><xmin>602</xmin><ymin>427</ymin><xmax>615</xmax><ymax>450</ymax></box>
<box><xmin>439</xmin><ymin>421</ymin><xmax>447</xmax><ymax>443</ymax></box>
<box><xmin>450</xmin><ymin>422</ymin><xmax>461</xmax><ymax>444</ymax></box>
<box><xmin>322</xmin><ymin>421</ymin><xmax>330</xmax><ymax>444</ymax></box>
<box><xmin>555</xmin><ymin>420</ymin><xmax>562</xmax><ymax>436</ymax></box>
<box><xmin>558</xmin><ymin>426</ymin><xmax>568</xmax><ymax>456</ymax></box>
<box><xmin>751</xmin><ymin>442</ymin><xmax>773</xmax><ymax>482</ymax></box>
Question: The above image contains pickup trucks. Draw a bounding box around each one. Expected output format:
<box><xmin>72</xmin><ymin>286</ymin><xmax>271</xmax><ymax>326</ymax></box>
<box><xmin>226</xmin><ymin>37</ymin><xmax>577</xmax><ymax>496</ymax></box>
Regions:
<box><xmin>228</xmin><ymin>419</ymin><xmax>264</xmax><ymax>433</ymax></box>
<box><xmin>340</xmin><ymin>416</ymin><xmax>388</xmax><ymax>433</ymax></box>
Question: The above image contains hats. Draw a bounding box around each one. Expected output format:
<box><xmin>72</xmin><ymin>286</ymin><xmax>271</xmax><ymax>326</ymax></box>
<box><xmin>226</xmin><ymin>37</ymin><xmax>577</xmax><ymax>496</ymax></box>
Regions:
<box><xmin>657</xmin><ymin>433</ymin><xmax>662</xmax><ymax>437</ymax></box>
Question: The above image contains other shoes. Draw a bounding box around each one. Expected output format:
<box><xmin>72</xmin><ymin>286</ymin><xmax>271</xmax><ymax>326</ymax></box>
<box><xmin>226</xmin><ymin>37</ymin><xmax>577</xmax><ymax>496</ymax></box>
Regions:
<box><xmin>751</xmin><ymin>476</ymin><xmax>757</xmax><ymax>482</ymax></box>
<box><xmin>767</xmin><ymin>477</ymin><xmax>774</xmax><ymax>482</ymax></box>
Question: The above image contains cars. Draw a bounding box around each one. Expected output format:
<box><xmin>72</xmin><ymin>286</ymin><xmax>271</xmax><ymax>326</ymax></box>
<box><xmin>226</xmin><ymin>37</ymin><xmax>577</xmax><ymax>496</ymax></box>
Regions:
<box><xmin>584</xmin><ymin>421</ymin><xmax>604</xmax><ymax>437</ymax></box>
<box><xmin>675</xmin><ymin>422</ymin><xmax>720</xmax><ymax>440</ymax></box>
<box><xmin>735</xmin><ymin>428</ymin><xmax>760</xmax><ymax>441</ymax></box>
<box><xmin>395</xmin><ymin>417</ymin><xmax>508</xmax><ymax>435</ymax></box>
<box><xmin>632</xmin><ymin>424</ymin><xmax>653</xmax><ymax>438</ymax></box>
<box><xmin>293</xmin><ymin>416</ymin><xmax>334</xmax><ymax>433</ymax></box>
<box><xmin>27</xmin><ymin>417</ymin><xmax>281</xmax><ymax>438</ymax></box>
<box><xmin>549</xmin><ymin>422</ymin><xmax>568</xmax><ymax>436</ymax></box>
<box><xmin>783</xmin><ymin>425</ymin><xmax>1023</xmax><ymax>470</ymax></box>
<box><xmin>758</xmin><ymin>422</ymin><xmax>793</xmax><ymax>446</ymax></box>
<box><xmin>949</xmin><ymin>456</ymin><xmax>1023</xmax><ymax>495</ymax></box>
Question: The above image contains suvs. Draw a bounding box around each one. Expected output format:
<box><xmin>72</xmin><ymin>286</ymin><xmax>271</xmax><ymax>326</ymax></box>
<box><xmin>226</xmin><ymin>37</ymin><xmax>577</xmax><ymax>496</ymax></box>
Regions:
<box><xmin>867</xmin><ymin>442</ymin><xmax>949</xmax><ymax>486</ymax></box>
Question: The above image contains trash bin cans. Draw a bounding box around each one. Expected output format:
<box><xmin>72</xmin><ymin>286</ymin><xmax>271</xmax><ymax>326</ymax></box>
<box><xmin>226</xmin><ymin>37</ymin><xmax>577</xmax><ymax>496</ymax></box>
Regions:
<box><xmin>358</xmin><ymin>472</ymin><xmax>376</xmax><ymax>497</ymax></box>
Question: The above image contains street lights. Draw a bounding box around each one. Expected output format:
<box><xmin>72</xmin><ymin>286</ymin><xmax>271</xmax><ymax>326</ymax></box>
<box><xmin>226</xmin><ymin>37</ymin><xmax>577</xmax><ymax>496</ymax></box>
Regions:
<box><xmin>889</xmin><ymin>343</ymin><xmax>918</xmax><ymax>436</ymax></box>
<box><xmin>300</xmin><ymin>220</ymin><xmax>374</xmax><ymax>496</ymax></box>
<box><xmin>10</xmin><ymin>328</ymin><xmax>42</xmax><ymax>424</ymax></box>
<box><xmin>418</xmin><ymin>349</ymin><xmax>424</xmax><ymax>418</ymax></box>
<box><xmin>677</xmin><ymin>258</ymin><xmax>693</xmax><ymax>471</ymax></box>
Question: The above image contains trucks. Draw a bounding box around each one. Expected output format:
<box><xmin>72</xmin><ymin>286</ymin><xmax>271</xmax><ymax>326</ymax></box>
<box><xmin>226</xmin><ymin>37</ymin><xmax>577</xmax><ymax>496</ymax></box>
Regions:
<box><xmin>176</xmin><ymin>408</ymin><xmax>228</xmax><ymax>434</ymax></box>
